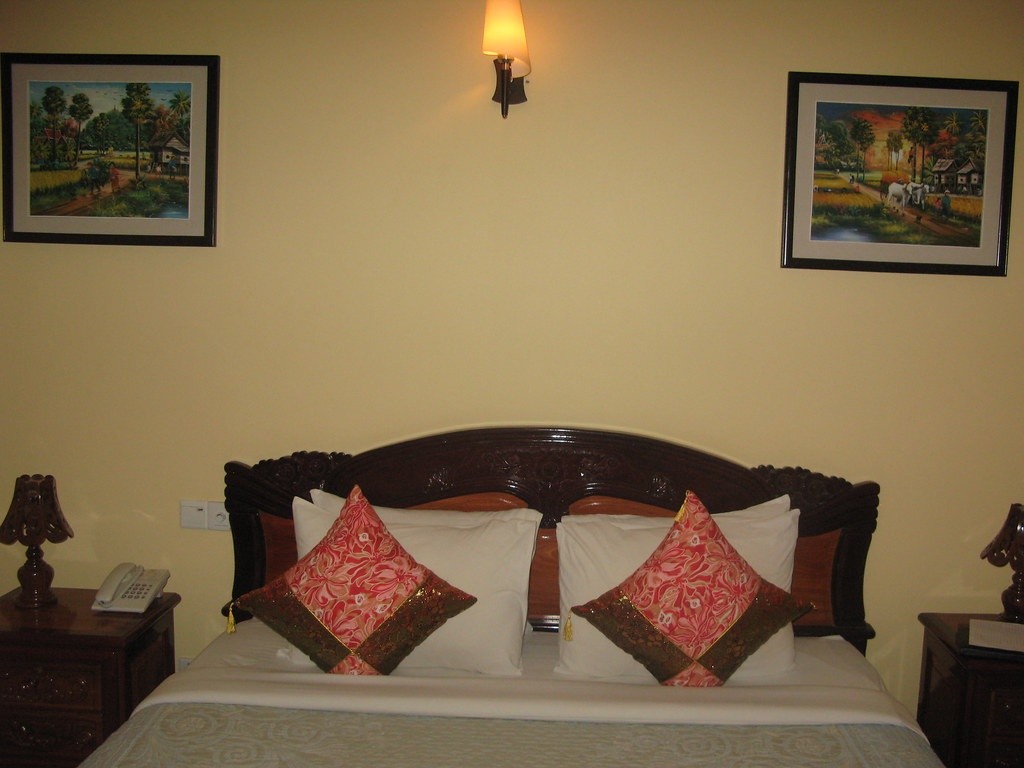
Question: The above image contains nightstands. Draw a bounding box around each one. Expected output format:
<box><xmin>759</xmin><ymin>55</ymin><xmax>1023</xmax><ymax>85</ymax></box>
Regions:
<box><xmin>0</xmin><ymin>586</ymin><xmax>182</xmax><ymax>768</ymax></box>
<box><xmin>915</xmin><ymin>612</ymin><xmax>1024</xmax><ymax>768</ymax></box>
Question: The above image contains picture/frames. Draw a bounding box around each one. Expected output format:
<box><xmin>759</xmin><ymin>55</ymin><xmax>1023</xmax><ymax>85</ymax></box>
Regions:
<box><xmin>780</xmin><ymin>71</ymin><xmax>1019</xmax><ymax>277</ymax></box>
<box><xmin>0</xmin><ymin>52</ymin><xmax>221</xmax><ymax>248</ymax></box>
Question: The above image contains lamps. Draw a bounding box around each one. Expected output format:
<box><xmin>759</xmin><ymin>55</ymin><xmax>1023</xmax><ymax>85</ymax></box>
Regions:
<box><xmin>979</xmin><ymin>503</ymin><xmax>1024</xmax><ymax>622</ymax></box>
<box><xmin>483</xmin><ymin>0</ymin><xmax>532</xmax><ymax>120</ymax></box>
<box><xmin>0</xmin><ymin>473</ymin><xmax>75</xmax><ymax>604</ymax></box>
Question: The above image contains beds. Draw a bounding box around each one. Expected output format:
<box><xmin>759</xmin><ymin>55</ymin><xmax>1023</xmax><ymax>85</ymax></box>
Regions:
<box><xmin>74</xmin><ymin>424</ymin><xmax>943</xmax><ymax>768</ymax></box>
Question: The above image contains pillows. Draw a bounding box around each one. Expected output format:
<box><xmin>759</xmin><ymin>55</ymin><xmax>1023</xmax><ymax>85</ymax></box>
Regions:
<box><xmin>223</xmin><ymin>485</ymin><xmax>477</xmax><ymax>675</ymax></box>
<box><xmin>555</xmin><ymin>493</ymin><xmax>801</xmax><ymax>687</ymax></box>
<box><xmin>292</xmin><ymin>489</ymin><xmax>544</xmax><ymax>683</ymax></box>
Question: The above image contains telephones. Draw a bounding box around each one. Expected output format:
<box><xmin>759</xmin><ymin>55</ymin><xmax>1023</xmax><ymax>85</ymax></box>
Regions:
<box><xmin>91</xmin><ymin>563</ymin><xmax>170</xmax><ymax>614</ymax></box>
